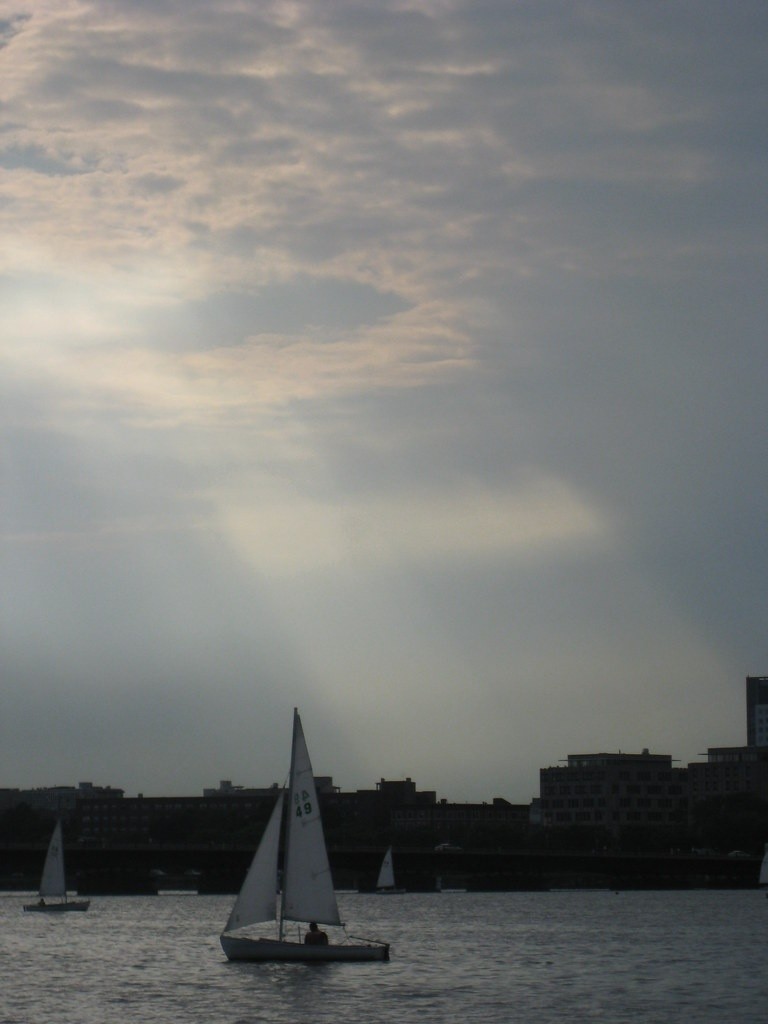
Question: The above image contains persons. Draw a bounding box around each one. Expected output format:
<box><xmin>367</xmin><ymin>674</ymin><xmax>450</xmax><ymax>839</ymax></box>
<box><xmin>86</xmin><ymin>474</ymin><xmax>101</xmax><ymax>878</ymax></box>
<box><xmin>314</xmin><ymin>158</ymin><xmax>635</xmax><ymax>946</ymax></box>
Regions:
<box><xmin>38</xmin><ymin>899</ymin><xmax>45</xmax><ymax>905</ymax></box>
<box><xmin>305</xmin><ymin>922</ymin><xmax>328</xmax><ymax>945</ymax></box>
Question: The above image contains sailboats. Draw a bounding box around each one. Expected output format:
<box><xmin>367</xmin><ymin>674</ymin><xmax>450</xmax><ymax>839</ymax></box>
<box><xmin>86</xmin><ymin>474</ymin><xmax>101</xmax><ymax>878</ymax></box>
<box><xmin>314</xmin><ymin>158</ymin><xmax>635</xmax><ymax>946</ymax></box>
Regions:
<box><xmin>22</xmin><ymin>820</ymin><xmax>92</xmax><ymax>912</ymax></box>
<box><xmin>219</xmin><ymin>706</ymin><xmax>391</xmax><ymax>963</ymax></box>
<box><xmin>373</xmin><ymin>843</ymin><xmax>407</xmax><ymax>895</ymax></box>
